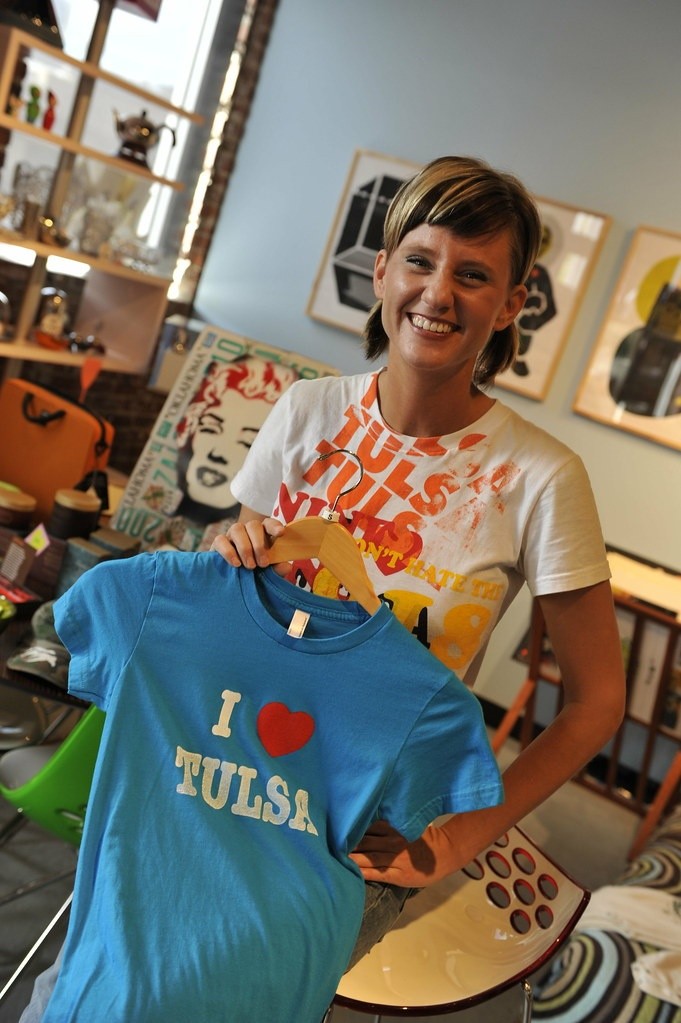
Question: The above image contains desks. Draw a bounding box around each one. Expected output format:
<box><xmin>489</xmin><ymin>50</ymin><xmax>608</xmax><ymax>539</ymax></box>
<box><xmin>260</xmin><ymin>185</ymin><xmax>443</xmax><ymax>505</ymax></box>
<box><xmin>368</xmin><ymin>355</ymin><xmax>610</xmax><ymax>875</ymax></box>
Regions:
<box><xmin>0</xmin><ymin>628</ymin><xmax>92</xmax><ymax>748</ymax></box>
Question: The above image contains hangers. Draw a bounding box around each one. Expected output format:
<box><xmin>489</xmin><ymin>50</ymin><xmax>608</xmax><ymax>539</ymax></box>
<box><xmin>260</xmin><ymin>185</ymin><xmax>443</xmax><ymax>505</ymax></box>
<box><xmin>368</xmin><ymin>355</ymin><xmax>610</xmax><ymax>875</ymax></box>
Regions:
<box><xmin>89</xmin><ymin>449</ymin><xmax>482</xmax><ymax>718</ymax></box>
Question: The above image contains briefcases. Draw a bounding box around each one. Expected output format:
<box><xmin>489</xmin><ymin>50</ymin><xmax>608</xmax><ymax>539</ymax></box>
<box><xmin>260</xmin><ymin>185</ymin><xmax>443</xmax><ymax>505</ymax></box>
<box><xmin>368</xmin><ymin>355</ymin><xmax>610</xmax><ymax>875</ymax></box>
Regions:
<box><xmin>1</xmin><ymin>378</ymin><xmax>117</xmax><ymax>531</ymax></box>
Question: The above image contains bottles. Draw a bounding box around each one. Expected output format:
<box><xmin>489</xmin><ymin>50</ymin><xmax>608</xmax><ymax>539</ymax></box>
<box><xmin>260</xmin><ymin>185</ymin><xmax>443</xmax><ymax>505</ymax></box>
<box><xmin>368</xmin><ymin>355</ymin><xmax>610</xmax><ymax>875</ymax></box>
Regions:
<box><xmin>38</xmin><ymin>285</ymin><xmax>68</xmax><ymax>340</ymax></box>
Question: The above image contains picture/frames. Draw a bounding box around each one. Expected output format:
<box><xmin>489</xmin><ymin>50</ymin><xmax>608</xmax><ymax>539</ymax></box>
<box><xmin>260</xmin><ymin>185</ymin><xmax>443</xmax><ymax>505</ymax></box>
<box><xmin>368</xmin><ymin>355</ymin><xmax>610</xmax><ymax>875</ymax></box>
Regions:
<box><xmin>306</xmin><ymin>148</ymin><xmax>423</xmax><ymax>336</ymax></box>
<box><xmin>473</xmin><ymin>199</ymin><xmax>606</xmax><ymax>402</ymax></box>
<box><xmin>574</xmin><ymin>224</ymin><xmax>680</xmax><ymax>450</ymax></box>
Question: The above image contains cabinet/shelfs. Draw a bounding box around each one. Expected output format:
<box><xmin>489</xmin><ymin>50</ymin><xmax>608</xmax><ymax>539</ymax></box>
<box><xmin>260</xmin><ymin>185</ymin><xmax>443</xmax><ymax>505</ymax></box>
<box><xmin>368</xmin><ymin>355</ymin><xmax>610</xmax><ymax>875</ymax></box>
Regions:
<box><xmin>0</xmin><ymin>33</ymin><xmax>203</xmax><ymax>378</ymax></box>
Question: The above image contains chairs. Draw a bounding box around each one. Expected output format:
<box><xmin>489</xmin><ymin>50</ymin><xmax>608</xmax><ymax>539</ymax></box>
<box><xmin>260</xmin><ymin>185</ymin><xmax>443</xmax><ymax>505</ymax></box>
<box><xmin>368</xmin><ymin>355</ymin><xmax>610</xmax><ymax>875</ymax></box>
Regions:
<box><xmin>0</xmin><ymin>704</ymin><xmax>106</xmax><ymax>848</ymax></box>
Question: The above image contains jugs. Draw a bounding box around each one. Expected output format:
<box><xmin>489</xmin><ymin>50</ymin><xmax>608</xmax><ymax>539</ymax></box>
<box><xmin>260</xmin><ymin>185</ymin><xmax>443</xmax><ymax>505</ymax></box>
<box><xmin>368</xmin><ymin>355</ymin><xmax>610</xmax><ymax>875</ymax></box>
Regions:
<box><xmin>107</xmin><ymin>104</ymin><xmax>178</xmax><ymax>166</ymax></box>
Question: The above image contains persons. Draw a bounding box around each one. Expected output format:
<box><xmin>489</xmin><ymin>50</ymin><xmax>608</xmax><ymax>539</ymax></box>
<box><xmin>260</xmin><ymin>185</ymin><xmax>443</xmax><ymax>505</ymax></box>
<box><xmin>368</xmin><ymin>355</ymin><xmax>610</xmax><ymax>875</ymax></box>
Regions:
<box><xmin>18</xmin><ymin>155</ymin><xmax>625</xmax><ymax>1023</ymax></box>
